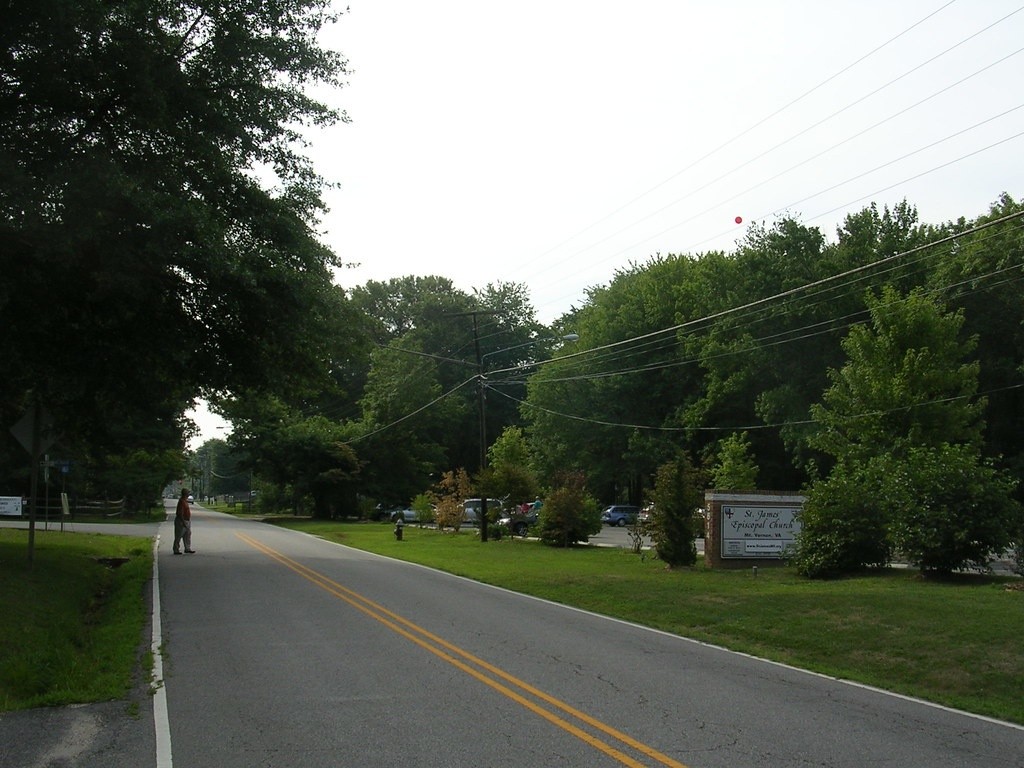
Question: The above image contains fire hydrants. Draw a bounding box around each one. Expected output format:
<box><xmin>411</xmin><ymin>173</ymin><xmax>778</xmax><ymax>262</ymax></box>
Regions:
<box><xmin>394</xmin><ymin>517</ymin><xmax>404</xmax><ymax>541</ymax></box>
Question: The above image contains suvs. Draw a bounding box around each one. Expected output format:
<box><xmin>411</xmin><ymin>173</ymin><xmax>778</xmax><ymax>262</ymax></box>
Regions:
<box><xmin>601</xmin><ymin>505</ymin><xmax>640</xmax><ymax>527</ymax></box>
<box><xmin>456</xmin><ymin>498</ymin><xmax>505</xmax><ymax>524</ymax></box>
<box><xmin>637</xmin><ymin>505</ymin><xmax>654</xmax><ymax>524</ymax></box>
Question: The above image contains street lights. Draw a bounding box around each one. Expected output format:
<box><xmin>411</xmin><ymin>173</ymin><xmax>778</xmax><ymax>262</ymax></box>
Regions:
<box><xmin>216</xmin><ymin>426</ymin><xmax>251</xmax><ymax>513</ymax></box>
<box><xmin>481</xmin><ymin>335</ymin><xmax>580</xmax><ymax>544</ymax></box>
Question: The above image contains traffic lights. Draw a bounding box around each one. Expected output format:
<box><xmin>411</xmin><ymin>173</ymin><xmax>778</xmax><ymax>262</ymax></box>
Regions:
<box><xmin>179</xmin><ymin>479</ymin><xmax>183</xmax><ymax>484</ymax></box>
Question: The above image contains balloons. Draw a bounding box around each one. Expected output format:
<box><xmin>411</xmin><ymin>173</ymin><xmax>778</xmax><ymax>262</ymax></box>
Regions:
<box><xmin>734</xmin><ymin>216</ymin><xmax>742</xmax><ymax>224</ymax></box>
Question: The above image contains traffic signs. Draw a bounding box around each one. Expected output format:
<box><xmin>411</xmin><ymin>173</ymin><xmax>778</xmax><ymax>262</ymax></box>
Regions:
<box><xmin>39</xmin><ymin>462</ymin><xmax>54</xmax><ymax>466</ymax></box>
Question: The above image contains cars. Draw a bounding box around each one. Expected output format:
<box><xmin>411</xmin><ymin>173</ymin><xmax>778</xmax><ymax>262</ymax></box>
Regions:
<box><xmin>186</xmin><ymin>495</ymin><xmax>195</xmax><ymax>504</ymax></box>
<box><xmin>389</xmin><ymin>504</ymin><xmax>439</xmax><ymax>523</ymax></box>
<box><xmin>495</xmin><ymin>503</ymin><xmax>543</xmax><ymax>537</ymax></box>
<box><xmin>162</xmin><ymin>494</ymin><xmax>182</xmax><ymax>499</ymax></box>
<box><xmin>249</xmin><ymin>491</ymin><xmax>256</xmax><ymax>497</ymax></box>
<box><xmin>692</xmin><ymin>508</ymin><xmax>706</xmax><ymax>519</ymax></box>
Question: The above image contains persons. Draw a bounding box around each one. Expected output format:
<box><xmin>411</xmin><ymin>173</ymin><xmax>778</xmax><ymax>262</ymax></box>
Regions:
<box><xmin>520</xmin><ymin>495</ymin><xmax>542</xmax><ymax>515</ymax></box>
<box><xmin>173</xmin><ymin>488</ymin><xmax>196</xmax><ymax>555</ymax></box>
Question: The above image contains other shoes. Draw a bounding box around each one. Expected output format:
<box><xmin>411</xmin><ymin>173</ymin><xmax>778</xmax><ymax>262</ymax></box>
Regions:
<box><xmin>174</xmin><ymin>552</ymin><xmax>182</xmax><ymax>554</ymax></box>
<box><xmin>184</xmin><ymin>550</ymin><xmax>195</xmax><ymax>553</ymax></box>
